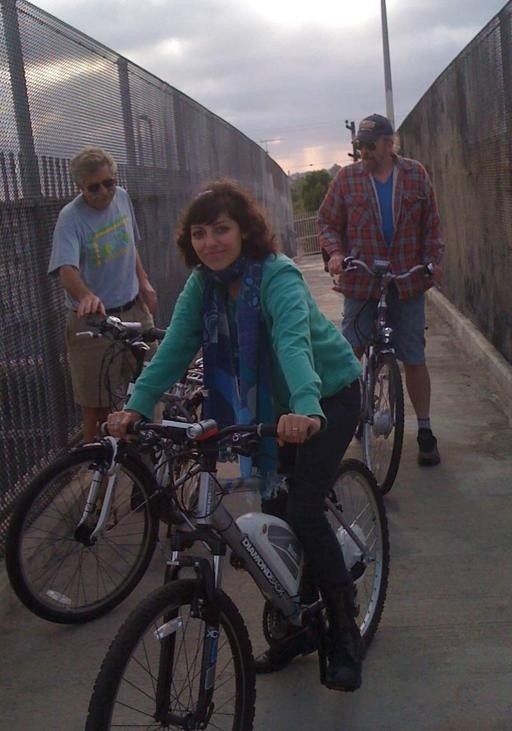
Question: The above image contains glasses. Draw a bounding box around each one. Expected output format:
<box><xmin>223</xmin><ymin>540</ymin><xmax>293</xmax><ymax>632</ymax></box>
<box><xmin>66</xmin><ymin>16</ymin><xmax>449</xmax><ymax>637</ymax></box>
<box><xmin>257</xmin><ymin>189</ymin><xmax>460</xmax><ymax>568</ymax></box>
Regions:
<box><xmin>85</xmin><ymin>178</ymin><xmax>114</xmax><ymax>193</ymax></box>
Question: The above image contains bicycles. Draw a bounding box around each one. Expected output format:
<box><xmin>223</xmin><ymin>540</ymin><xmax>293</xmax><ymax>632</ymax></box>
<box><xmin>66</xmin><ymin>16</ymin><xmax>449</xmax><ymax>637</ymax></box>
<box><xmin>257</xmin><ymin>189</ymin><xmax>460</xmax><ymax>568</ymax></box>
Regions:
<box><xmin>324</xmin><ymin>253</ymin><xmax>436</xmax><ymax>495</ymax></box>
<box><xmin>3</xmin><ymin>310</ymin><xmax>235</xmax><ymax>624</ymax></box>
<box><xmin>84</xmin><ymin>414</ymin><xmax>392</xmax><ymax>731</ymax></box>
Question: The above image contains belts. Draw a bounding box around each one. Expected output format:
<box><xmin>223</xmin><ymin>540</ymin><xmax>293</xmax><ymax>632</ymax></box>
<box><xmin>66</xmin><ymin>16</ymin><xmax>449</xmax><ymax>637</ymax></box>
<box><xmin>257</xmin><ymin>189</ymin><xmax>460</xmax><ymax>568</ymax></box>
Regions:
<box><xmin>73</xmin><ymin>293</ymin><xmax>140</xmax><ymax>315</ymax></box>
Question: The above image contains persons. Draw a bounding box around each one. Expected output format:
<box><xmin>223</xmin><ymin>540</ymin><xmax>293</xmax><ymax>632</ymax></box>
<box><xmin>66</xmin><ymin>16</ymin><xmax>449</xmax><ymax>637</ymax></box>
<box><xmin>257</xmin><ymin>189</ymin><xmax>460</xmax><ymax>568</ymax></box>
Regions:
<box><xmin>316</xmin><ymin>113</ymin><xmax>446</xmax><ymax>467</ymax></box>
<box><xmin>47</xmin><ymin>147</ymin><xmax>159</xmax><ymax>515</ymax></box>
<box><xmin>108</xmin><ymin>178</ymin><xmax>365</xmax><ymax>692</ymax></box>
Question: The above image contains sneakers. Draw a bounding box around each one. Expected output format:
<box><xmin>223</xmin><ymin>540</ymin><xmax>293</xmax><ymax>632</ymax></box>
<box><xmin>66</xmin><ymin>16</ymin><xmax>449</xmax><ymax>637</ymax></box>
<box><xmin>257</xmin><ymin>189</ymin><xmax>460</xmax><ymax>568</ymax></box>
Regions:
<box><xmin>415</xmin><ymin>431</ymin><xmax>439</xmax><ymax>467</ymax></box>
<box><xmin>253</xmin><ymin>612</ymin><xmax>328</xmax><ymax>673</ymax></box>
<box><xmin>327</xmin><ymin>629</ymin><xmax>368</xmax><ymax>692</ymax></box>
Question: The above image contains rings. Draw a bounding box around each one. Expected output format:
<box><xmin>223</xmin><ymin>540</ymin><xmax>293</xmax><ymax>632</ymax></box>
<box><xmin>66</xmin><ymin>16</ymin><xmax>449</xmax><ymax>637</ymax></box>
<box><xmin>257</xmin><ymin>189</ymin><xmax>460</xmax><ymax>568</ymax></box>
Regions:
<box><xmin>292</xmin><ymin>427</ymin><xmax>297</xmax><ymax>431</ymax></box>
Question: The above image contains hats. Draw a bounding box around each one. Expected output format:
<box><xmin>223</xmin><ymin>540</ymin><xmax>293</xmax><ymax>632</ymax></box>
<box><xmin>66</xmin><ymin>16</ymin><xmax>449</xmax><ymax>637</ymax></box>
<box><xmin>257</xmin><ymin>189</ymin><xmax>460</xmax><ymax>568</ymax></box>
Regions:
<box><xmin>352</xmin><ymin>114</ymin><xmax>394</xmax><ymax>144</ymax></box>
